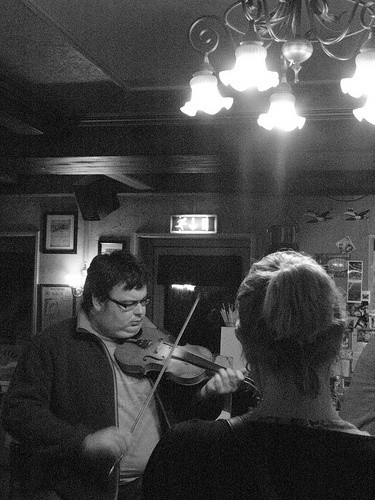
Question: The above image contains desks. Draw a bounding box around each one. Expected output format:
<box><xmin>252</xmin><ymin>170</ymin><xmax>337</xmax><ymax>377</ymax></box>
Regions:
<box><xmin>221</xmin><ymin>327</ymin><xmax>250</xmax><ymax>371</ymax></box>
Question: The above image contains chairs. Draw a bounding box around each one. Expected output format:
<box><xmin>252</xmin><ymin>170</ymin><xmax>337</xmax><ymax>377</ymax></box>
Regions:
<box><xmin>332</xmin><ymin>359</ymin><xmax>351</xmax><ymax>395</ymax></box>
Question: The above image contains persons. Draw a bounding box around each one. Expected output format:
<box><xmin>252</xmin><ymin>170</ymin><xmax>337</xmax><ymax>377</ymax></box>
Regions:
<box><xmin>337</xmin><ymin>335</ymin><xmax>375</xmax><ymax>438</ymax></box>
<box><xmin>0</xmin><ymin>248</ymin><xmax>246</xmax><ymax>500</ymax></box>
<box><xmin>142</xmin><ymin>249</ymin><xmax>375</xmax><ymax>500</ymax></box>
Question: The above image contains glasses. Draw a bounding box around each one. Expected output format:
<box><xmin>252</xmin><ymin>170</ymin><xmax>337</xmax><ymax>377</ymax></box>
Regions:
<box><xmin>106</xmin><ymin>295</ymin><xmax>152</xmax><ymax>312</ymax></box>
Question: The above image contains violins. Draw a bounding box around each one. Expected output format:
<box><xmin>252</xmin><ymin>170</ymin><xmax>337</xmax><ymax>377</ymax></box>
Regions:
<box><xmin>113</xmin><ymin>326</ymin><xmax>264</xmax><ymax>404</ymax></box>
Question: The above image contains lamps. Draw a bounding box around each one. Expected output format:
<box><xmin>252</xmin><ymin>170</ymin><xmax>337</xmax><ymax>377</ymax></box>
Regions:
<box><xmin>180</xmin><ymin>0</ymin><xmax>375</xmax><ymax>133</ymax></box>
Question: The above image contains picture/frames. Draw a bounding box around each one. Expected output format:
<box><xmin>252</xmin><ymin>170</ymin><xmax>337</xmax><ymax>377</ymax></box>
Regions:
<box><xmin>42</xmin><ymin>211</ymin><xmax>78</xmax><ymax>254</ymax></box>
<box><xmin>37</xmin><ymin>284</ymin><xmax>77</xmax><ymax>336</ymax></box>
<box><xmin>98</xmin><ymin>240</ymin><xmax>125</xmax><ymax>257</ymax></box>
